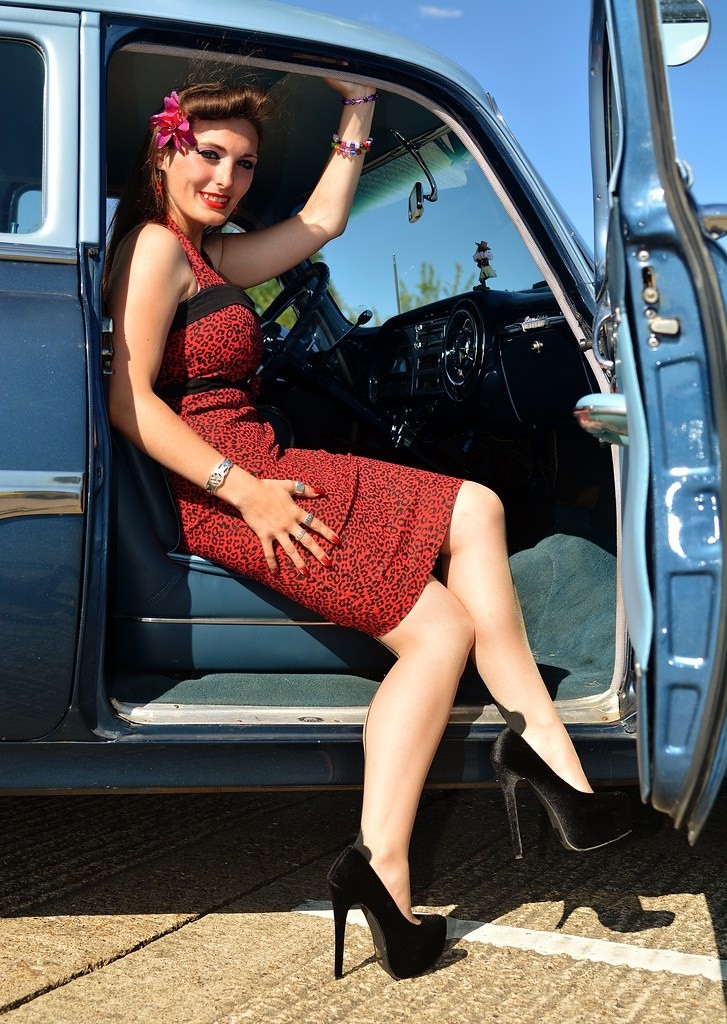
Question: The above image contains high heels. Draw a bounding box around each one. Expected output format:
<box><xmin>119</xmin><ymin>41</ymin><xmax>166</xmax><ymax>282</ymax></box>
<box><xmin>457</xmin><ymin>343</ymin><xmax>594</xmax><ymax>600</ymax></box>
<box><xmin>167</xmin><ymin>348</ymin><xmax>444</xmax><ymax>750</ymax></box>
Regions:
<box><xmin>490</xmin><ymin>726</ymin><xmax>634</xmax><ymax>860</ymax></box>
<box><xmin>326</xmin><ymin>845</ymin><xmax>447</xmax><ymax>981</ymax></box>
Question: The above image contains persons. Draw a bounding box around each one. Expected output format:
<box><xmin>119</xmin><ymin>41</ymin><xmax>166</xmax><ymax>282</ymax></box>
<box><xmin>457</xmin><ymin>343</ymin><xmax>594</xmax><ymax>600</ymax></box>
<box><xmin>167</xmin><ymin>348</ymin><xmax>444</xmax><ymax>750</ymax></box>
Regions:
<box><xmin>101</xmin><ymin>77</ymin><xmax>636</xmax><ymax>980</ymax></box>
<box><xmin>473</xmin><ymin>241</ymin><xmax>497</xmax><ymax>286</ymax></box>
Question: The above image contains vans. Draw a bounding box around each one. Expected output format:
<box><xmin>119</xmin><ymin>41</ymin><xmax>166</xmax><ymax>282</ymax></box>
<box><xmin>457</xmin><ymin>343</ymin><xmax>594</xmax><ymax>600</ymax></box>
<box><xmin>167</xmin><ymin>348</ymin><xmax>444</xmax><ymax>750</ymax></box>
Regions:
<box><xmin>1</xmin><ymin>1</ymin><xmax>727</xmax><ymax>852</ymax></box>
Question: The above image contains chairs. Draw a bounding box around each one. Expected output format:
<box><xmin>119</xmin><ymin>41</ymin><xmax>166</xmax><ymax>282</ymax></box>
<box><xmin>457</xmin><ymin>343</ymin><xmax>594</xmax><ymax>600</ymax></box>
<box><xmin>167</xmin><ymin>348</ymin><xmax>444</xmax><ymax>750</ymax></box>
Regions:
<box><xmin>104</xmin><ymin>373</ymin><xmax>368</xmax><ymax>674</ymax></box>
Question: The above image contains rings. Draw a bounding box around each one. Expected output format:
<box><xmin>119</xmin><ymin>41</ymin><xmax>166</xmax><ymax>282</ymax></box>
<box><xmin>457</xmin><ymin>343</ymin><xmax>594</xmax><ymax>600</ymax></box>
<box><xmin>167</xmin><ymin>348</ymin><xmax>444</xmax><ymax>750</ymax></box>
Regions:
<box><xmin>296</xmin><ymin>530</ymin><xmax>306</xmax><ymax>541</ymax></box>
<box><xmin>305</xmin><ymin>513</ymin><xmax>314</xmax><ymax>524</ymax></box>
<box><xmin>296</xmin><ymin>482</ymin><xmax>304</xmax><ymax>496</ymax></box>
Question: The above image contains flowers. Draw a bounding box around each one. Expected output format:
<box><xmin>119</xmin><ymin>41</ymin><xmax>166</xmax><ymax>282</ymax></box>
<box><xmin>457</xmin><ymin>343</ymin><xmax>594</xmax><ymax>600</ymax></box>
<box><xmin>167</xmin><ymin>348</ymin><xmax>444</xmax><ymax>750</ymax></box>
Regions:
<box><xmin>149</xmin><ymin>90</ymin><xmax>201</xmax><ymax>158</ymax></box>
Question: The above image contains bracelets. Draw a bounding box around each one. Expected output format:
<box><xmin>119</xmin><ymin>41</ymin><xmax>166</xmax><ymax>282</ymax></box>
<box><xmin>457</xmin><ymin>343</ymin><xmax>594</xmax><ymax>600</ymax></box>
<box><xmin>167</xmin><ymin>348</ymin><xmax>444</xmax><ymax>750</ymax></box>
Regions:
<box><xmin>204</xmin><ymin>459</ymin><xmax>234</xmax><ymax>495</ymax></box>
<box><xmin>330</xmin><ymin>130</ymin><xmax>373</xmax><ymax>155</ymax></box>
<box><xmin>342</xmin><ymin>93</ymin><xmax>380</xmax><ymax>104</ymax></box>
